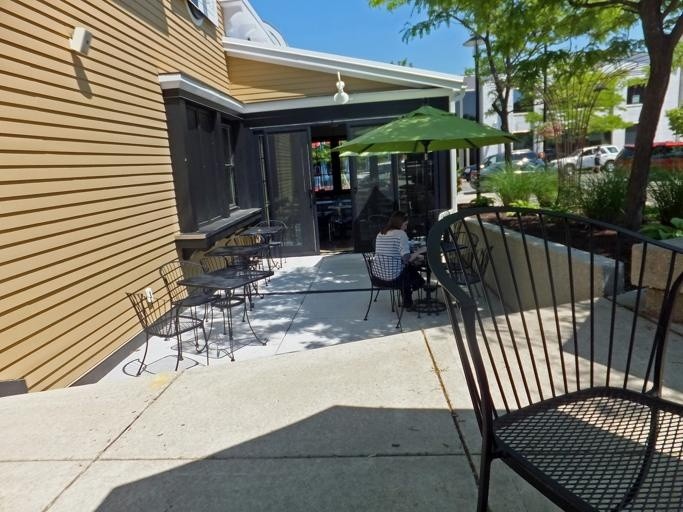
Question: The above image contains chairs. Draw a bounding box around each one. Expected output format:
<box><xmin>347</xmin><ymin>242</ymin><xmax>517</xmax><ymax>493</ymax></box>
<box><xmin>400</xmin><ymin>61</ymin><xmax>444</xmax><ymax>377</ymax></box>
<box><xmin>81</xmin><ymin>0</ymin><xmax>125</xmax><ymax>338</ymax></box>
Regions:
<box><xmin>427</xmin><ymin>206</ymin><xmax>683</xmax><ymax>511</ymax></box>
<box><xmin>124</xmin><ymin>220</ymin><xmax>288</xmax><ymax>378</ymax></box>
<box><xmin>360</xmin><ymin>209</ymin><xmax>495</xmax><ymax>328</ymax></box>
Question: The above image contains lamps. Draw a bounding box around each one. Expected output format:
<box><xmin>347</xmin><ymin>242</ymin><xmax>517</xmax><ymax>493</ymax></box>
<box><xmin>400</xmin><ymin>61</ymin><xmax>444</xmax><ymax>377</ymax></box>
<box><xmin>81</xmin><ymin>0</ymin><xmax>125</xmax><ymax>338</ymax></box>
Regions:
<box><xmin>330</xmin><ymin>70</ymin><xmax>349</xmax><ymax>105</ymax></box>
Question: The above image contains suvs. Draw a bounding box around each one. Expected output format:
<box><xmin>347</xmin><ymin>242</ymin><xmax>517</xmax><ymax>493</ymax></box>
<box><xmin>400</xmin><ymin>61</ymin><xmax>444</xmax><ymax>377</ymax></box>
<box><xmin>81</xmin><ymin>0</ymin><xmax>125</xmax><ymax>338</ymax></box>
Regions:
<box><xmin>457</xmin><ymin>138</ymin><xmax>682</xmax><ymax>194</ymax></box>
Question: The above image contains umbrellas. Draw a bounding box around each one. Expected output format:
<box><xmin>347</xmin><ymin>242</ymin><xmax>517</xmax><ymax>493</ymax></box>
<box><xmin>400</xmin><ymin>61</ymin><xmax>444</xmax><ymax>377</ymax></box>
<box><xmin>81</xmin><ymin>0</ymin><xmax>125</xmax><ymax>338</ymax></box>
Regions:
<box><xmin>325</xmin><ymin>103</ymin><xmax>522</xmax><ymax>287</ymax></box>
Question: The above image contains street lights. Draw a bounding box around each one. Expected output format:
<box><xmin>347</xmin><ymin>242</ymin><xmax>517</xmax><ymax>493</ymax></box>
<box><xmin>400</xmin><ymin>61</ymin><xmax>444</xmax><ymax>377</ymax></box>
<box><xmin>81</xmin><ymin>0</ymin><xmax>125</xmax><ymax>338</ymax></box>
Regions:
<box><xmin>461</xmin><ymin>29</ymin><xmax>492</xmax><ymax>206</ymax></box>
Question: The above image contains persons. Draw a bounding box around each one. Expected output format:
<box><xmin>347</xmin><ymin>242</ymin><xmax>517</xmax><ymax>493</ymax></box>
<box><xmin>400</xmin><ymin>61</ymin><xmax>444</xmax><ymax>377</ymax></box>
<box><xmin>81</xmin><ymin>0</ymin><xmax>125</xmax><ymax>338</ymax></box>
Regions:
<box><xmin>369</xmin><ymin>210</ymin><xmax>440</xmax><ymax>309</ymax></box>
<box><xmin>537</xmin><ymin>151</ymin><xmax>547</xmax><ymax>170</ymax></box>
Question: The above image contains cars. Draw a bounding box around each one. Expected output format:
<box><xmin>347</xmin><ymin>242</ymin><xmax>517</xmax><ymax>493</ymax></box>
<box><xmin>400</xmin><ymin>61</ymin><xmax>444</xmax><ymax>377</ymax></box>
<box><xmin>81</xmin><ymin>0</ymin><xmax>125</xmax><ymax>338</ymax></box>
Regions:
<box><xmin>312</xmin><ymin>153</ymin><xmax>408</xmax><ymax>193</ymax></box>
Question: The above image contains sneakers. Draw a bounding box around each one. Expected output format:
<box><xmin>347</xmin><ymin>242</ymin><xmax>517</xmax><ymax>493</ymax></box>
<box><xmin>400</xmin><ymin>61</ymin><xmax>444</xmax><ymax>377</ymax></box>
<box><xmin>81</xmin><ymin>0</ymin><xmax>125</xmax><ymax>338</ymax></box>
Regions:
<box><xmin>422</xmin><ymin>280</ymin><xmax>438</xmax><ymax>290</ymax></box>
<box><xmin>407</xmin><ymin>302</ymin><xmax>416</xmax><ymax>312</ymax></box>
